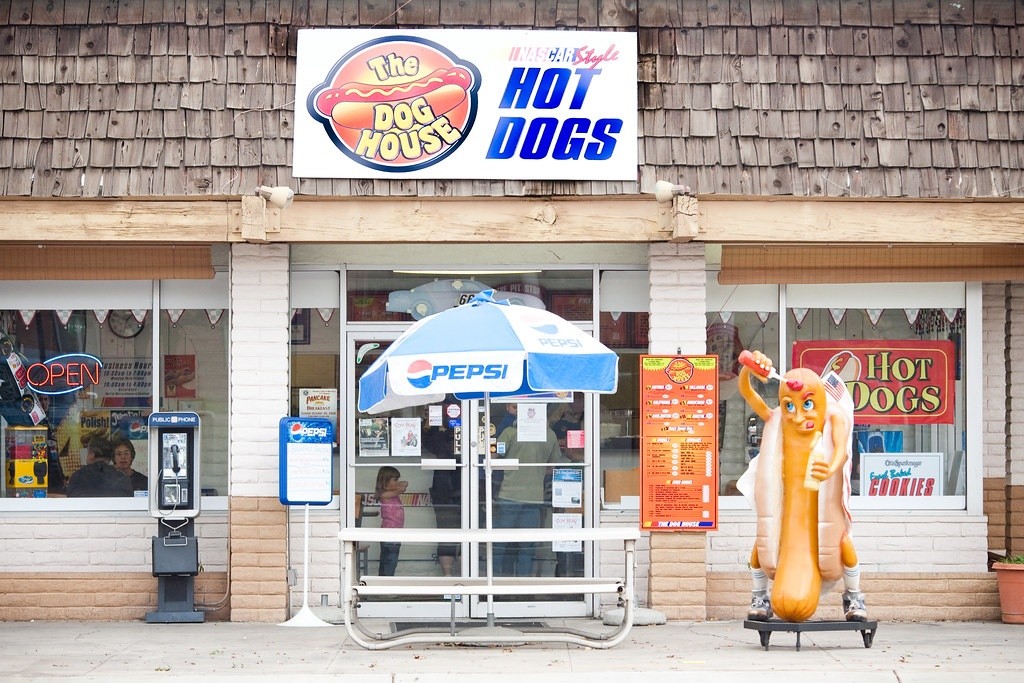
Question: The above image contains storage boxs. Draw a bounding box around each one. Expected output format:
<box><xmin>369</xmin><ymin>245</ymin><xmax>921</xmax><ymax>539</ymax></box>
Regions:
<box><xmin>603</xmin><ymin>468</ymin><xmax>639</xmax><ymax>502</ymax></box>
<box><xmin>611</xmin><ymin>409</ymin><xmax>639</xmax><ymax>436</ymax></box>
<box><xmin>726</xmin><ymin>479</ymin><xmax>742</xmax><ymax>496</ymax></box>
<box><xmin>4</xmin><ymin>425</ymin><xmax>48</xmax><ymax>498</ymax></box>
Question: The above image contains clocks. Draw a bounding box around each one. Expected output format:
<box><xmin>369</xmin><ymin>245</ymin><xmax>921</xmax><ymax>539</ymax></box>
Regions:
<box><xmin>108</xmin><ymin>309</ymin><xmax>146</xmax><ymax>339</ymax></box>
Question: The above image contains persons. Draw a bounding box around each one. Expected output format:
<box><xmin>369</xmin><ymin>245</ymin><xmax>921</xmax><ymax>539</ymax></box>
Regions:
<box><xmin>66</xmin><ymin>438</ymin><xmax>134</xmax><ymax>498</ymax></box>
<box><xmin>375</xmin><ymin>466</ymin><xmax>408</xmax><ymax>576</ymax></box>
<box><xmin>36</xmin><ymin>395</ymin><xmax>49</xmax><ymax>413</ymax></box>
<box><xmin>418</xmin><ymin>397</ymin><xmax>588</xmax><ymax>602</ymax></box>
<box><xmin>110</xmin><ymin>436</ymin><xmax>148</xmax><ymax>491</ymax></box>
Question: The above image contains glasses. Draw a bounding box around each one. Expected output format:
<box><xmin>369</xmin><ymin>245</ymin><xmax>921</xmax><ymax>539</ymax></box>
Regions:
<box><xmin>39</xmin><ymin>398</ymin><xmax>50</xmax><ymax>401</ymax></box>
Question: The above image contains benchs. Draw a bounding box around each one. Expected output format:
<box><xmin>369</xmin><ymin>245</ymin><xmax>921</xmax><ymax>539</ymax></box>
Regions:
<box><xmin>358</xmin><ymin>574</ymin><xmax>623</xmax><ymax>584</ymax></box>
<box><xmin>351</xmin><ymin>584</ymin><xmax>625</xmax><ymax>597</ymax></box>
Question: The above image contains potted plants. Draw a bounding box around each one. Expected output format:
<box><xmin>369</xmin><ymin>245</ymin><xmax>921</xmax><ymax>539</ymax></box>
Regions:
<box><xmin>991</xmin><ymin>553</ymin><xmax>1024</xmax><ymax>624</ymax></box>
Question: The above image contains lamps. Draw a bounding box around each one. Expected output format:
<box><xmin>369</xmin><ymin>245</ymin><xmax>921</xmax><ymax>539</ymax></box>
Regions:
<box><xmin>654</xmin><ymin>179</ymin><xmax>690</xmax><ymax>203</ymax></box>
<box><xmin>255</xmin><ymin>185</ymin><xmax>294</xmax><ymax>210</ymax></box>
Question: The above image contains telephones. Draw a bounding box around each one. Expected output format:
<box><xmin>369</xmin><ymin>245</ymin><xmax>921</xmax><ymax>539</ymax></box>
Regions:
<box><xmin>162</xmin><ymin>433</ymin><xmax>187</xmax><ymax>479</ymax></box>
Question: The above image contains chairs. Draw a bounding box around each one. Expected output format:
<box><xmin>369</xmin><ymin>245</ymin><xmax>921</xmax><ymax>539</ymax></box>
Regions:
<box><xmin>355</xmin><ymin>493</ymin><xmax>371</xmax><ymax>578</ymax></box>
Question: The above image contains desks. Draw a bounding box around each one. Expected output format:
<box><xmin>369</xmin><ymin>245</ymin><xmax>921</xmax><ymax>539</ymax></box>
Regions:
<box><xmin>335</xmin><ymin>526</ymin><xmax>642</xmax><ymax>651</ymax></box>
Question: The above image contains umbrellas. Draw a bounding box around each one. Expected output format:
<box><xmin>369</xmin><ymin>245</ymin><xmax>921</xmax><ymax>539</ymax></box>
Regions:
<box><xmin>357</xmin><ymin>288</ymin><xmax>620</xmax><ymax>628</ymax></box>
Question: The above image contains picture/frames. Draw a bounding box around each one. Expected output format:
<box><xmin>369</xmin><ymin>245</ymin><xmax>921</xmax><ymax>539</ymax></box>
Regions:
<box><xmin>543</xmin><ymin>289</ymin><xmax>630</xmax><ymax>348</ymax></box>
<box><xmin>347</xmin><ymin>290</ymin><xmax>403</xmax><ymax>349</ymax></box>
<box><xmin>631</xmin><ymin>312</ymin><xmax>648</xmax><ymax>348</ymax></box>
<box><xmin>290</xmin><ymin>309</ymin><xmax>309</xmax><ymax>345</ymax></box>
